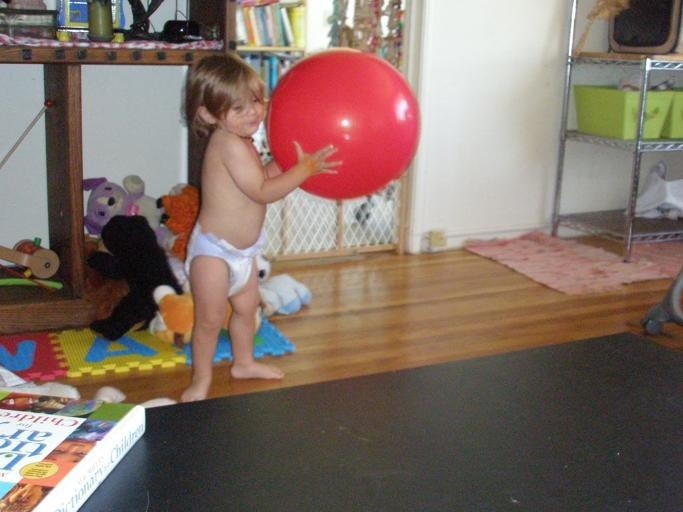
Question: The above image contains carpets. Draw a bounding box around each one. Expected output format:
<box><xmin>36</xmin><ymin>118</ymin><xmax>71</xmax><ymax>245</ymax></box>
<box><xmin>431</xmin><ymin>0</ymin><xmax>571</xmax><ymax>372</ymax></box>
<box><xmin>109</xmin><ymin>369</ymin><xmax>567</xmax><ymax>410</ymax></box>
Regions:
<box><xmin>462</xmin><ymin>230</ymin><xmax>672</xmax><ymax>298</ymax></box>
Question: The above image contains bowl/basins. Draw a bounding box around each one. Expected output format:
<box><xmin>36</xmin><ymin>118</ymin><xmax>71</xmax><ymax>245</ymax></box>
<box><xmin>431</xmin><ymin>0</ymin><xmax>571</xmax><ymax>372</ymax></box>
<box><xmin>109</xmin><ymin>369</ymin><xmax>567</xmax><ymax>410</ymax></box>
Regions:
<box><xmin>56</xmin><ymin>31</ymin><xmax>76</xmax><ymax>42</ymax></box>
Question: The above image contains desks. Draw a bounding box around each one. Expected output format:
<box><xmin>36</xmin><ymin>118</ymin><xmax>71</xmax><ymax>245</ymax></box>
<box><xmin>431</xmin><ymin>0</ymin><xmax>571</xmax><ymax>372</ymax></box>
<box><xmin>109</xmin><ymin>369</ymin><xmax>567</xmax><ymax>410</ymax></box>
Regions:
<box><xmin>75</xmin><ymin>331</ymin><xmax>682</xmax><ymax>511</ymax></box>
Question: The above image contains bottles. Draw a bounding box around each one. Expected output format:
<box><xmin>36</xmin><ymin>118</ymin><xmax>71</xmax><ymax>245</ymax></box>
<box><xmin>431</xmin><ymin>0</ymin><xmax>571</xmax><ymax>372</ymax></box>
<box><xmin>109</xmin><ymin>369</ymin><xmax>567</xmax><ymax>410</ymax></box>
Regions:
<box><xmin>87</xmin><ymin>0</ymin><xmax>115</xmax><ymax>42</ymax></box>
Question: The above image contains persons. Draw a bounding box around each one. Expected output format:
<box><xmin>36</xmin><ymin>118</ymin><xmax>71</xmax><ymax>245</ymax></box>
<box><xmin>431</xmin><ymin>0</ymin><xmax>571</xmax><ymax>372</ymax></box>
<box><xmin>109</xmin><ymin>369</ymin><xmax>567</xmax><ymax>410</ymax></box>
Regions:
<box><xmin>172</xmin><ymin>48</ymin><xmax>346</xmax><ymax>405</ymax></box>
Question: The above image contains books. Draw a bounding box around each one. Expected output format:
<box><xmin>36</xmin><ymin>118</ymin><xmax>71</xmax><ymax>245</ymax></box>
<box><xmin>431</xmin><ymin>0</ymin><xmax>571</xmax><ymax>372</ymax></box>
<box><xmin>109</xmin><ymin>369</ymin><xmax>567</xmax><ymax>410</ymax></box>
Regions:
<box><xmin>231</xmin><ymin>1</ymin><xmax>306</xmax><ymax>45</ymax></box>
<box><xmin>0</xmin><ymin>390</ymin><xmax>147</xmax><ymax>512</ymax></box>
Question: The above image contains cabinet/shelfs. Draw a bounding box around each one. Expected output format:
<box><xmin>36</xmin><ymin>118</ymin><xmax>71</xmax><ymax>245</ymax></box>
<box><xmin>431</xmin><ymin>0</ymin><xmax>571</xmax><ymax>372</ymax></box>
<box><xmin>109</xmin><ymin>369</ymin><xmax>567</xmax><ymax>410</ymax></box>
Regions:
<box><xmin>549</xmin><ymin>0</ymin><xmax>683</xmax><ymax>263</ymax></box>
<box><xmin>0</xmin><ymin>0</ymin><xmax>237</xmax><ymax>336</ymax></box>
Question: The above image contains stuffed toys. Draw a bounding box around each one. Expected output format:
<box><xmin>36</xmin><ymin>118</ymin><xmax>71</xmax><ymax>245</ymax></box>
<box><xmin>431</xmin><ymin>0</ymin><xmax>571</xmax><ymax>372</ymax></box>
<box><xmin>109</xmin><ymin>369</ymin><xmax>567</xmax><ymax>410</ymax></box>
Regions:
<box><xmin>78</xmin><ymin>217</ymin><xmax>274</xmax><ymax>345</ymax></box>
<box><xmin>78</xmin><ymin>173</ymin><xmax>313</xmax><ymax>344</ymax></box>
<box><xmin>82</xmin><ymin>175</ymin><xmax>172</xmax><ymax>250</ymax></box>
<box><xmin>156</xmin><ymin>183</ymin><xmax>201</xmax><ymax>262</ymax></box>
<box><xmin>256</xmin><ymin>247</ymin><xmax>312</xmax><ymax>317</ymax></box>
